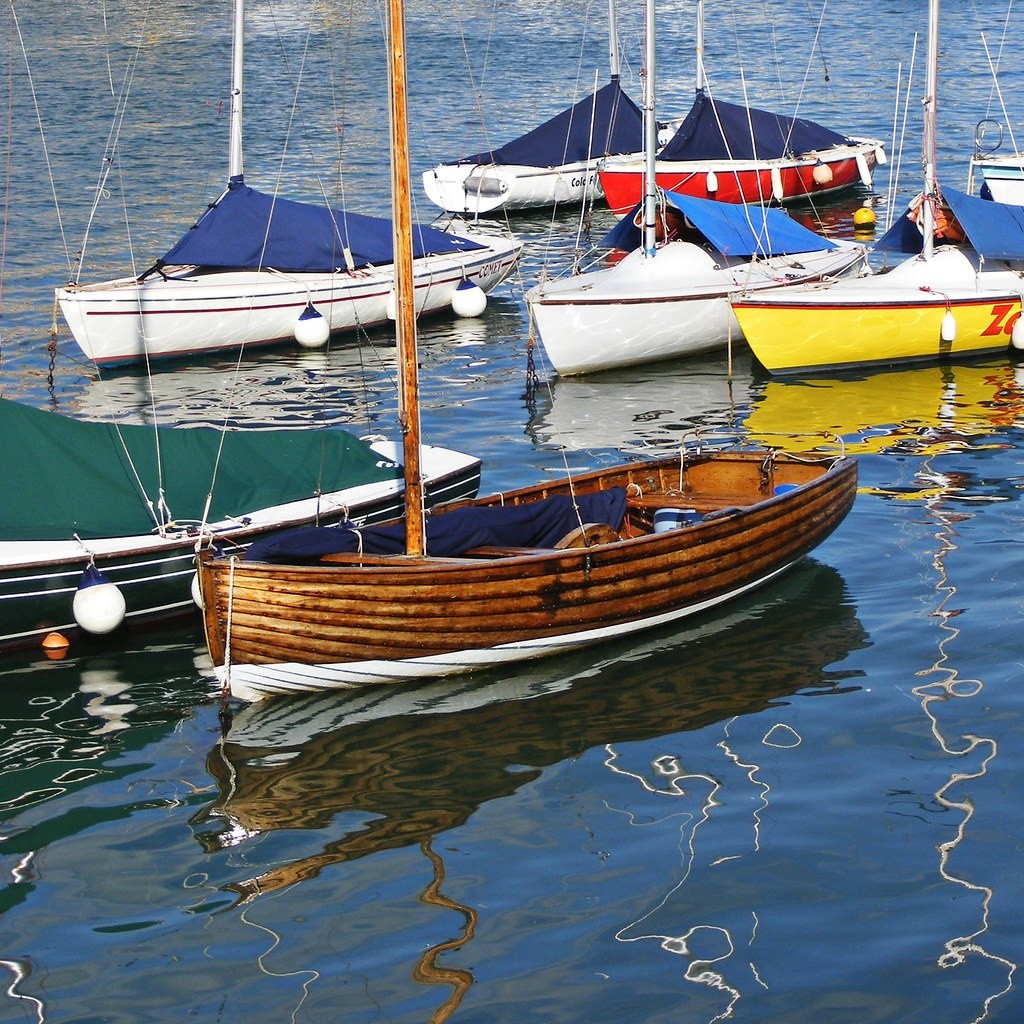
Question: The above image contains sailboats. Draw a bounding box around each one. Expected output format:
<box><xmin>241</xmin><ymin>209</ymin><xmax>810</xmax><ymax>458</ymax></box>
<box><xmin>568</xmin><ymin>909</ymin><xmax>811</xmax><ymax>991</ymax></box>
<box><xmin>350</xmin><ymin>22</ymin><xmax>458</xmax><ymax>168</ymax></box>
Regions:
<box><xmin>196</xmin><ymin>2</ymin><xmax>864</xmax><ymax>702</ymax></box>
<box><xmin>54</xmin><ymin>1</ymin><xmax>526</xmax><ymax>369</ymax></box>
<box><xmin>414</xmin><ymin>0</ymin><xmax>1020</xmax><ymax>375</ymax></box>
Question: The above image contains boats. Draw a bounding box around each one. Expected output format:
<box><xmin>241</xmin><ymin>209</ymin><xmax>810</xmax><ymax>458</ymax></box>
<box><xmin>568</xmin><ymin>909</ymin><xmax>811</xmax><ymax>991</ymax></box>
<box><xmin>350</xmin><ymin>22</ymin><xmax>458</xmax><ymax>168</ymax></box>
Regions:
<box><xmin>191</xmin><ymin>565</ymin><xmax>868</xmax><ymax>856</ymax></box>
<box><xmin>0</xmin><ymin>395</ymin><xmax>487</xmax><ymax>662</ymax></box>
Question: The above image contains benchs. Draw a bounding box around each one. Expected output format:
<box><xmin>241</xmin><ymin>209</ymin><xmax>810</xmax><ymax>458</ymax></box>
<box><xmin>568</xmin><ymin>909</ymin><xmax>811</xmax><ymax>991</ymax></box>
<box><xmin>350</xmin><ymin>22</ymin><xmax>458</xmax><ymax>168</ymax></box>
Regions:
<box><xmin>320</xmin><ymin>552</ymin><xmax>479</xmax><ymax>566</ymax></box>
<box><xmin>469</xmin><ymin>545</ymin><xmax>560</xmax><ymax>556</ymax></box>
<box><xmin>626</xmin><ymin>493</ymin><xmax>767</xmax><ymax>513</ymax></box>
